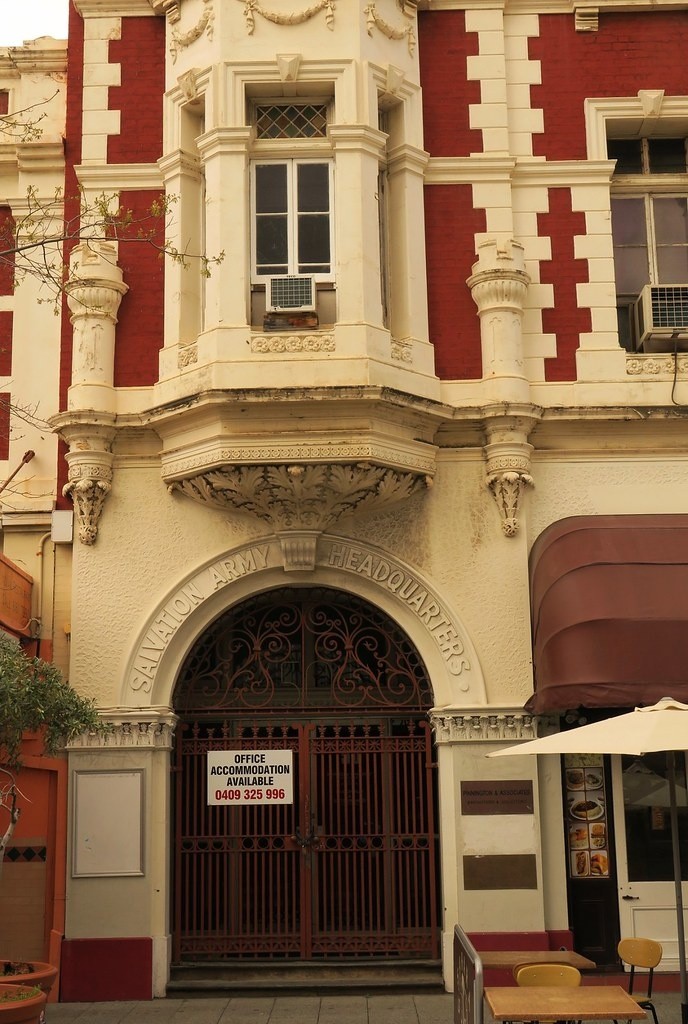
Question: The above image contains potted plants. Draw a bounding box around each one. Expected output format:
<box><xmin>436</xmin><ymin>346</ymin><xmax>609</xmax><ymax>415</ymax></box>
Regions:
<box><xmin>0</xmin><ymin>960</ymin><xmax>58</xmax><ymax>1024</ymax></box>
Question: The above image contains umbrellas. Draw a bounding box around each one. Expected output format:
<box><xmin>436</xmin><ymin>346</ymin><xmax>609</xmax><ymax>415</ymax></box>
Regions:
<box><xmin>625</xmin><ymin>779</ymin><xmax>688</xmax><ymax>807</ymax></box>
<box><xmin>485</xmin><ymin>697</ymin><xmax>688</xmax><ymax>1024</ymax></box>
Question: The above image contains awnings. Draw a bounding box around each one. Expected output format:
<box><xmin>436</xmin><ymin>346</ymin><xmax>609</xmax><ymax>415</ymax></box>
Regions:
<box><xmin>523</xmin><ymin>513</ymin><xmax>688</xmax><ymax>713</ymax></box>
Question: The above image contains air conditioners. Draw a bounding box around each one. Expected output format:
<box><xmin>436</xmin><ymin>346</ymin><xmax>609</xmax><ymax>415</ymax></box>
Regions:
<box><xmin>265</xmin><ymin>275</ymin><xmax>318</xmax><ymax>313</ymax></box>
<box><xmin>633</xmin><ymin>284</ymin><xmax>688</xmax><ymax>342</ymax></box>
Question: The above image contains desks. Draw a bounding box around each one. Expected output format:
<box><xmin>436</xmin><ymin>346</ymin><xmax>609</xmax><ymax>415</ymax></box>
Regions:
<box><xmin>477</xmin><ymin>951</ymin><xmax>596</xmax><ymax>970</ymax></box>
<box><xmin>484</xmin><ymin>986</ymin><xmax>648</xmax><ymax>1024</ymax></box>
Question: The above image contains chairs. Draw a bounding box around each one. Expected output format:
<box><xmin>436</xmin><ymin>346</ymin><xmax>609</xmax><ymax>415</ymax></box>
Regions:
<box><xmin>513</xmin><ymin>937</ymin><xmax>662</xmax><ymax>1024</ymax></box>
